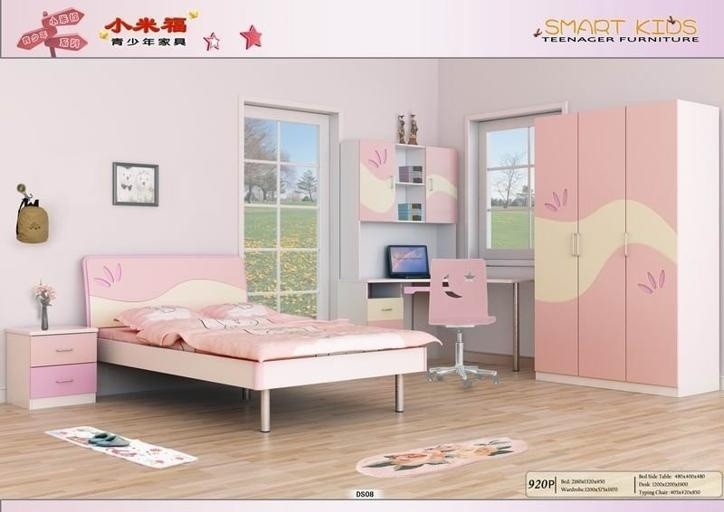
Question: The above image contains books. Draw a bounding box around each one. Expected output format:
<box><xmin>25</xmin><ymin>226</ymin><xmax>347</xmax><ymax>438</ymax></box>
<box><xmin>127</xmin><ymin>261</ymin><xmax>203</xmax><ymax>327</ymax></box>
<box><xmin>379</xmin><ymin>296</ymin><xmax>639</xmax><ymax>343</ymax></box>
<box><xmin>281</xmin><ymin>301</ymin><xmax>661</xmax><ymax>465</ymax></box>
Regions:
<box><xmin>398</xmin><ymin>166</ymin><xmax>423</xmax><ymax>184</ymax></box>
<box><xmin>399</xmin><ymin>202</ymin><xmax>422</xmax><ymax>221</ymax></box>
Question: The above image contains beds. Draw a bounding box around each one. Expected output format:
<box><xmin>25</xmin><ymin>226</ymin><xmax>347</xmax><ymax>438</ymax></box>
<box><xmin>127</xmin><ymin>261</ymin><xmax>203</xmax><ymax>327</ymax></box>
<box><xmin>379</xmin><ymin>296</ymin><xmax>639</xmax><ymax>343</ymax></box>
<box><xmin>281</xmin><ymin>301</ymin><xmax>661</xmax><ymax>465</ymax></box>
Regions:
<box><xmin>82</xmin><ymin>255</ymin><xmax>442</xmax><ymax>438</ymax></box>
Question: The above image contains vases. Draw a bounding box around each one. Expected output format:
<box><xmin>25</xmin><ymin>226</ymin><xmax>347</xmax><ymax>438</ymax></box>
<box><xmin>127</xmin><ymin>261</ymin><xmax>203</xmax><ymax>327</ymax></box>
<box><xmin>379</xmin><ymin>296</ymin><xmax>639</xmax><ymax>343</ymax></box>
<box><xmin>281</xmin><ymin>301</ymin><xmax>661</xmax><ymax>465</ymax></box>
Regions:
<box><xmin>42</xmin><ymin>304</ymin><xmax>48</xmax><ymax>330</ymax></box>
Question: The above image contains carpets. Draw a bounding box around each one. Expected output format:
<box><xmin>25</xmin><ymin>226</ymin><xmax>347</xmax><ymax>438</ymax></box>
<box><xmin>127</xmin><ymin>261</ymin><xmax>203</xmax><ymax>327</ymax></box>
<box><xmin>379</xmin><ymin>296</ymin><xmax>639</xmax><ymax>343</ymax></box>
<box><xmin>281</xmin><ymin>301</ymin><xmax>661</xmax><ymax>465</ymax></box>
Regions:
<box><xmin>356</xmin><ymin>435</ymin><xmax>528</xmax><ymax>479</ymax></box>
<box><xmin>44</xmin><ymin>425</ymin><xmax>198</xmax><ymax>469</ymax></box>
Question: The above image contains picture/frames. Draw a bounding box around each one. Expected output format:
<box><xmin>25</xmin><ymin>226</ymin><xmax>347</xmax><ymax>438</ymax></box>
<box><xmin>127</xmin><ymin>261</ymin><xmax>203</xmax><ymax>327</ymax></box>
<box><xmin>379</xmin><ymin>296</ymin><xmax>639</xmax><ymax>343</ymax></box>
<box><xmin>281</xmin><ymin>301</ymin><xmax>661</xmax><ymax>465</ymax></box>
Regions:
<box><xmin>113</xmin><ymin>163</ymin><xmax>160</xmax><ymax>207</ymax></box>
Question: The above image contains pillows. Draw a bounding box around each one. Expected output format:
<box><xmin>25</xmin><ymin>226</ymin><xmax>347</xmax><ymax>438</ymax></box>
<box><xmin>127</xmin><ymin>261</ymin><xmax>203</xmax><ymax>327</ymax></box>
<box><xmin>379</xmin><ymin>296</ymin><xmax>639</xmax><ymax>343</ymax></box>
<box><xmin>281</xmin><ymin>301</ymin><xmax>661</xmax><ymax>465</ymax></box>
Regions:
<box><xmin>114</xmin><ymin>301</ymin><xmax>279</xmax><ymax>334</ymax></box>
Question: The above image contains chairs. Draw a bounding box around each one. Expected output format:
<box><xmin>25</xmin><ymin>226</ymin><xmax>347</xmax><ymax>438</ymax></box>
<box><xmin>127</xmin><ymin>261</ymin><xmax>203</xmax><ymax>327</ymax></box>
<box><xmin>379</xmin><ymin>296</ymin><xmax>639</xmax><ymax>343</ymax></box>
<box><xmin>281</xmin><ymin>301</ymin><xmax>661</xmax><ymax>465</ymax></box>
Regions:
<box><xmin>426</xmin><ymin>258</ymin><xmax>500</xmax><ymax>389</ymax></box>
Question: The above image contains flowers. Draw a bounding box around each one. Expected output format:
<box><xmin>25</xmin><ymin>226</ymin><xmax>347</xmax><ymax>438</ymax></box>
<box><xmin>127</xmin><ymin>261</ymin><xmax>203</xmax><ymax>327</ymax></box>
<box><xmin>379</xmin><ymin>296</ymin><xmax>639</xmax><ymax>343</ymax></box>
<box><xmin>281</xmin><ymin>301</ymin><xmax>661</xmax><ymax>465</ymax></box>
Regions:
<box><xmin>33</xmin><ymin>279</ymin><xmax>56</xmax><ymax>308</ymax></box>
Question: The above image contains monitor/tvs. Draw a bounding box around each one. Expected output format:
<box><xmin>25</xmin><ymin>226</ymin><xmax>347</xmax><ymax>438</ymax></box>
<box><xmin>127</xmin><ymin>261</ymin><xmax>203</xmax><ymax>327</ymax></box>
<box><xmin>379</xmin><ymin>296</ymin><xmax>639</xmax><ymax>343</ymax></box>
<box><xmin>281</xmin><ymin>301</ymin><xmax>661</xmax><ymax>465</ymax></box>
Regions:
<box><xmin>385</xmin><ymin>245</ymin><xmax>429</xmax><ymax>278</ymax></box>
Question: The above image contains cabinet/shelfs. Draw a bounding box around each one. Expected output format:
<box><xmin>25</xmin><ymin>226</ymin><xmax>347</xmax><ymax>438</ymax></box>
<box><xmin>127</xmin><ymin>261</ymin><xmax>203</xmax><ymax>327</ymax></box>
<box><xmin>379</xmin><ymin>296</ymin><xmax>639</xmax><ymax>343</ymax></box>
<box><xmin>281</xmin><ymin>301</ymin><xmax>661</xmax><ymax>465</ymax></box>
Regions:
<box><xmin>532</xmin><ymin>100</ymin><xmax>720</xmax><ymax>397</ymax></box>
<box><xmin>341</xmin><ymin>137</ymin><xmax>458</xmax><ymax>225</ymax></box>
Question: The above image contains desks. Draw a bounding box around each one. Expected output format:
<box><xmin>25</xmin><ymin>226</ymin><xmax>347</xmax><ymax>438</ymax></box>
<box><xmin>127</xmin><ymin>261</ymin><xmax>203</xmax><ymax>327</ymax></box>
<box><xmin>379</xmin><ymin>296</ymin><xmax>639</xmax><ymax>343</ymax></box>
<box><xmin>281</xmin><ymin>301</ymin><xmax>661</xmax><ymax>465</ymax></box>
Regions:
<box><xmin>335</xmin><ymin>277</ymin><xmax>533</xmax><ymax>372</ymax></box>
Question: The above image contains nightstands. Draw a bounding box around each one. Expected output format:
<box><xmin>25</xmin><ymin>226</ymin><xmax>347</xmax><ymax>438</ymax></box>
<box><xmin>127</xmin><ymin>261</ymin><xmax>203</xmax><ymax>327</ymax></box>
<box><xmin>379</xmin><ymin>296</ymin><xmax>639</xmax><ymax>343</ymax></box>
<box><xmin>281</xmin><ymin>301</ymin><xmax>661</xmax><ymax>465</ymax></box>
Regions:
<box><xmin>5</xmin><ymin>325</ymin><xmax>99</xmax><ymax>411</ymax></box>
